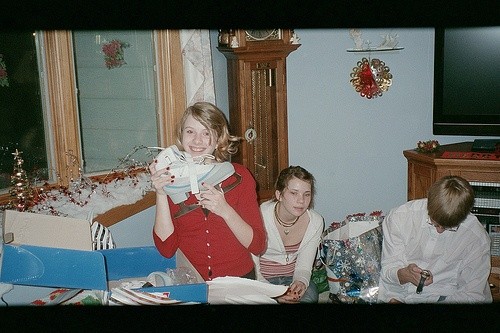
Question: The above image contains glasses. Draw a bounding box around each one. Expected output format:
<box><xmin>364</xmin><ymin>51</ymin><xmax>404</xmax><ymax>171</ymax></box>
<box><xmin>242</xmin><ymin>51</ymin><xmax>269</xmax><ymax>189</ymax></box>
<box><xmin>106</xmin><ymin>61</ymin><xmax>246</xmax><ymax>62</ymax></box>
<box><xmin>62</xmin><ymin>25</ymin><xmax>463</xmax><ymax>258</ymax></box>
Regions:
<box><xmin>426</xmin><ymin>215</ymin><xmax>460</xmax><ymax>232</ymax></box>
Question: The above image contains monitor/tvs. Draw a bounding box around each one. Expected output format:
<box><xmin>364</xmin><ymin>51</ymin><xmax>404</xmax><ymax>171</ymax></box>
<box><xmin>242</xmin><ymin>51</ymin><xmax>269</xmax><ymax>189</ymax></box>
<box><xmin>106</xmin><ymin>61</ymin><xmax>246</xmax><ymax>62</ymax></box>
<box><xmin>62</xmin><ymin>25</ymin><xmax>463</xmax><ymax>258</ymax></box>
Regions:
<box><xmin>433</xmin><ymin>26</ymin><xmax>500</xmax><ymax>136</ymax></box>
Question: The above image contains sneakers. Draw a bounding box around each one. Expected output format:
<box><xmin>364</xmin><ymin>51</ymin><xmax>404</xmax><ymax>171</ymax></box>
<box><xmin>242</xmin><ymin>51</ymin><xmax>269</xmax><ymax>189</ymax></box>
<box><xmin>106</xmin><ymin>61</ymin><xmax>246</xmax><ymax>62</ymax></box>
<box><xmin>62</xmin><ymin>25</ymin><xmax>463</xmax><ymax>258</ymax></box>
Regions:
<box><xmin>148</xmin><ymin>145</ymin><xmax>242</xmax><ymax>219</ymax></box>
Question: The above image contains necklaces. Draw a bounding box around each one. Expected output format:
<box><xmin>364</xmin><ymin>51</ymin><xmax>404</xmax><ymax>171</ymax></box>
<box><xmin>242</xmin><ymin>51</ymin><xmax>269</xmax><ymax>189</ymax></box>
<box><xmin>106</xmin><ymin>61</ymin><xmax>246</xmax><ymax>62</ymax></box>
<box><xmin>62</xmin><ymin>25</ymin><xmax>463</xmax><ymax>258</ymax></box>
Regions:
<box><xmin>274</xmin><ymin>202</ymin><xmax>300</xmax><ymax>235</ymax></box>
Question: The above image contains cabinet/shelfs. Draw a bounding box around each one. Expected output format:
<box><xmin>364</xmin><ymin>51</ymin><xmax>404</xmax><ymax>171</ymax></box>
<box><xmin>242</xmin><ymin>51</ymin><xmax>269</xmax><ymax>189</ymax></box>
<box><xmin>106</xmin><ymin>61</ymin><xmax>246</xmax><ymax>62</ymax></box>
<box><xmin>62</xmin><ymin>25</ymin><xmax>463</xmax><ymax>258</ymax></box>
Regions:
<box><xmin>403</xmin><ymin>141</ymin><xmax>500</xmax><ymax>284</ymax></box>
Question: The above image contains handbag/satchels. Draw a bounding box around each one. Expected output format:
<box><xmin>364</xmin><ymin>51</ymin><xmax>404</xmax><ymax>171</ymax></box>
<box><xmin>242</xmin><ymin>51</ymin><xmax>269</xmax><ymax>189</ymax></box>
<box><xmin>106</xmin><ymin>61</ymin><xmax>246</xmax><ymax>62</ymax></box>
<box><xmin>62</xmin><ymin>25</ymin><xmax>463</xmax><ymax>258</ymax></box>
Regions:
<box><xmin>318</xmin><ymin>215</ymin><xmax>386</xmax><ymax>302</ymax></box>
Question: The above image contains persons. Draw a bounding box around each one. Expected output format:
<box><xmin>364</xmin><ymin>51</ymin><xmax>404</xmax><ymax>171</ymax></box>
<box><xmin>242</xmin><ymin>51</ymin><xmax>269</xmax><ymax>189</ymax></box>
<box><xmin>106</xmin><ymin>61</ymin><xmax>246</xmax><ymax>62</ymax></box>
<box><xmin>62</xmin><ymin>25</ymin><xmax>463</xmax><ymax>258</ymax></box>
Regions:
<box><xmin>251</xmin><ymin>165</ymin><xmax>324</xmax><ymax>303</ymax></box>
<box><xmin>377</xmin><ymin>175</ymin><xmax>493</xmax><ymax>303</ymax></box>
<box><xmin>149</xmin><ymin>102</ymin><xmax>268</xmax><ymax>280</ymax></box>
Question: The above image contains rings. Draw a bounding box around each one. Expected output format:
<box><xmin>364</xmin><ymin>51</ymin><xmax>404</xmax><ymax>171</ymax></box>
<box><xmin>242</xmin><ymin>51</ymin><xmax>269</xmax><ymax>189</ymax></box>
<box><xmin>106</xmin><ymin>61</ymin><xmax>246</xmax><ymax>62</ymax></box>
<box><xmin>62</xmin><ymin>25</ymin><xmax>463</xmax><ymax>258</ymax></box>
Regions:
<box><xmin>201</xmin><ymin>204</ymin><xmax>205</xmax><ymax>208</ymax></box>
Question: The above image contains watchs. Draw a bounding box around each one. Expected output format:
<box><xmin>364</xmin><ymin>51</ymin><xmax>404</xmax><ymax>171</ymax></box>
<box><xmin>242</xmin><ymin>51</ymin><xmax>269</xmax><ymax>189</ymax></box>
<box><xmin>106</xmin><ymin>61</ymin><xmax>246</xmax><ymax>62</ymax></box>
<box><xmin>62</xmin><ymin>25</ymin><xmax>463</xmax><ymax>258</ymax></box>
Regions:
<box><xmin>416</xmin><ymin>269</ymin><xmax>431</xmax><ymax>294</ymax></box>
